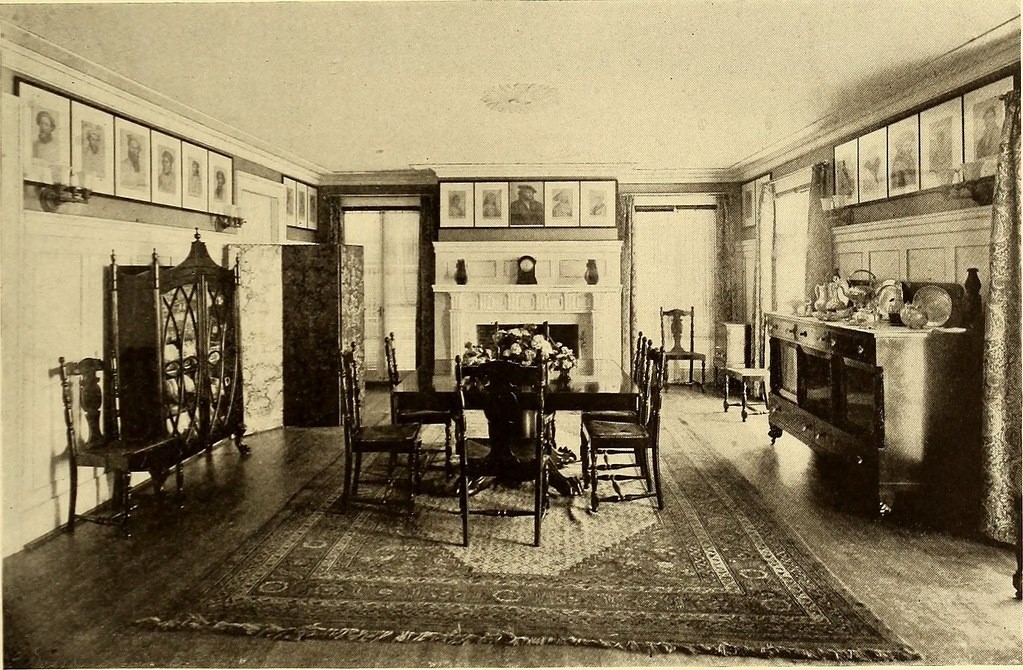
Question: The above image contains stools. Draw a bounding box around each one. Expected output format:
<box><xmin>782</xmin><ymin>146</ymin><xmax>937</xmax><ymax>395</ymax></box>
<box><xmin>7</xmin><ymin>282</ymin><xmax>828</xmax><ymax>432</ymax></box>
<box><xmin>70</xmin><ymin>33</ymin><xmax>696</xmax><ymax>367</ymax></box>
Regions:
<box><xmin>721</xmin><ymin>368</ymin><xmax>770</xmax><ymax>422</ymax></box>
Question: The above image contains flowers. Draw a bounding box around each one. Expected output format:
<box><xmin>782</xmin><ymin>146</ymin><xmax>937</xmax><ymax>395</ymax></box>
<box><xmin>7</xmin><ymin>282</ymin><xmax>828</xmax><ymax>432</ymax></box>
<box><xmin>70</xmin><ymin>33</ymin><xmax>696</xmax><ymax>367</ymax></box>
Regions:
<box><xmin>462</xmin><ymin>323</ymin><xmax>578</xmax><ymax>386</ymax></box>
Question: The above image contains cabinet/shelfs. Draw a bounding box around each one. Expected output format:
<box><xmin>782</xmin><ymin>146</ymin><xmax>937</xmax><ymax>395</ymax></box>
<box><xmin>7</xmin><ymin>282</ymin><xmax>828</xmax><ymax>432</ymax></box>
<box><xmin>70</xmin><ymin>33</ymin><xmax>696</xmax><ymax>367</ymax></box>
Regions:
<box><xmin>762</xmin><ymin>313</ymin><xmax>983</xmax><ymax>519</ymax></box>
<box><xmin>110</xmin><ymin>227</ymin><xmax>253</xmax><ymax>499</ymax></box>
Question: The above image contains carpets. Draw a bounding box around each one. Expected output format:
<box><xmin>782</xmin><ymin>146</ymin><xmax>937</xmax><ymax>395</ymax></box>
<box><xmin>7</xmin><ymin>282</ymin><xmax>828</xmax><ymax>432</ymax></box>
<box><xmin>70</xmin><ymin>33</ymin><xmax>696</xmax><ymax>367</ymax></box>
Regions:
<box><xmin>134</xmin><ymin>418</ymin><xmax>924</xmax><ymax>663</ymax></box>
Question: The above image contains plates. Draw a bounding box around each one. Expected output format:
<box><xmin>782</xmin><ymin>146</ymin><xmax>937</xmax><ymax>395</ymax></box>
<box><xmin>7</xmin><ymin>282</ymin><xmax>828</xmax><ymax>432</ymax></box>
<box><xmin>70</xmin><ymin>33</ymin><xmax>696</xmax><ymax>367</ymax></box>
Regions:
<box><xmin>912</xmin><ymin>285</ymin><xmax>952</xmax><ymax>327</ymax></box>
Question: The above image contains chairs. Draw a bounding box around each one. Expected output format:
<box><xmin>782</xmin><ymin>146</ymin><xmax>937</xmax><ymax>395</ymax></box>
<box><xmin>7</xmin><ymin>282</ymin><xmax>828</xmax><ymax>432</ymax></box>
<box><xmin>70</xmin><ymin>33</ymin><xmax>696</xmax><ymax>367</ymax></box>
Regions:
<box><xmin>385</xmin><ymin>332</ymin><xmax>461</xmax><ymax>466</ymax></box>
<box><xmin>580</xmin><ymin>306</ymin><xmax>705</xmax><ymax>512</ymax></box>
<box><xmin>338</xmin><ymin>342</ymin><xmax>422</xmax><ymax>517</ymax></box>
<box><xmin>59</xmin><ymin>349</ymin><xmax>184</xmax><ymax>539</ymax></box>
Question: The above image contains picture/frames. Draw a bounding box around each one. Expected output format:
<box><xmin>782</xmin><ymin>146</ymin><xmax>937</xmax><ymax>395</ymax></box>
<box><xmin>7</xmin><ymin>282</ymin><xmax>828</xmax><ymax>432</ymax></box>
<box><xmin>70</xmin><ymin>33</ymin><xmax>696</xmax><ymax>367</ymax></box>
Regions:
<box><xmin>440</xmin><ymin>179</ymin><xmax>619</xmax><ymax>229</ymax></box>
<box><xmin>282</xmin><ymin>174</ymin><xmax>318</xmax><ymax>231</ymax></box>
<box><xmin>833</xmin><ymin>74</ymin><xmax>1017</xmax><ymax>208</ymax></box>
<box><xmin>740</xmin><ymin>172</ymin><xmax>772</xmax><ymax>229</ymax></box>
<box><xmin>15</xmin><ymin>76</ymin><xmax>234</xmax><ymax>216</ymax></box>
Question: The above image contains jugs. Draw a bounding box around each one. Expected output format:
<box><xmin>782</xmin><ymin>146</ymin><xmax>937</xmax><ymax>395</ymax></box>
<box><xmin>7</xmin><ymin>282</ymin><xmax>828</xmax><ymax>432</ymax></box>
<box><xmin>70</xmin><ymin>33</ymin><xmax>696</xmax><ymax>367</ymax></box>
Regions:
<box><xmin>838</xmin><ymin>269</ymin><xmax>877</xmax><ymax>302</ymax></box>
<box><xmin>826</xmin><ymin>282</ymin><xmax>845</xmax><ymax>313</ymax></box>
<box><xmin>814</xmin><ymin>282</ymin><xmax>828</xmax><ymax>314</ymax></box>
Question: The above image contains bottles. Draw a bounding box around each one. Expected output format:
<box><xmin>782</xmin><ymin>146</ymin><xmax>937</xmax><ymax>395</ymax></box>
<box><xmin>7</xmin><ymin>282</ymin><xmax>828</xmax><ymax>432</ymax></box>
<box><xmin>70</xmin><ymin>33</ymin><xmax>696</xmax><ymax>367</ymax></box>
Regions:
<box><xmin>584</xmin><ymin>259</ymin><xmax>599</xmax><ymax>285</ymax></box>
<box><xmin>455</xmin><ymin>258</ymin><xmax>467</xmax><ymax>285</ymax></box>
<box><xmin>963</xmin><ymin>268</ymin><xmax>982</xmax><ymax>327</ymax></box>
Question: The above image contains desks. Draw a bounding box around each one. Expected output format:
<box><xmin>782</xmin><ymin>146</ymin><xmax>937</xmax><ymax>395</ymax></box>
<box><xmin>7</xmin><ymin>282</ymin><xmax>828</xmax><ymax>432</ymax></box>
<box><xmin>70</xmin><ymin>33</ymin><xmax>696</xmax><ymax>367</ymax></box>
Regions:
<box><xmin>390</xmin><ymin>358</ymin><xmax>644</xmax><ymax>498</ymax></box>
